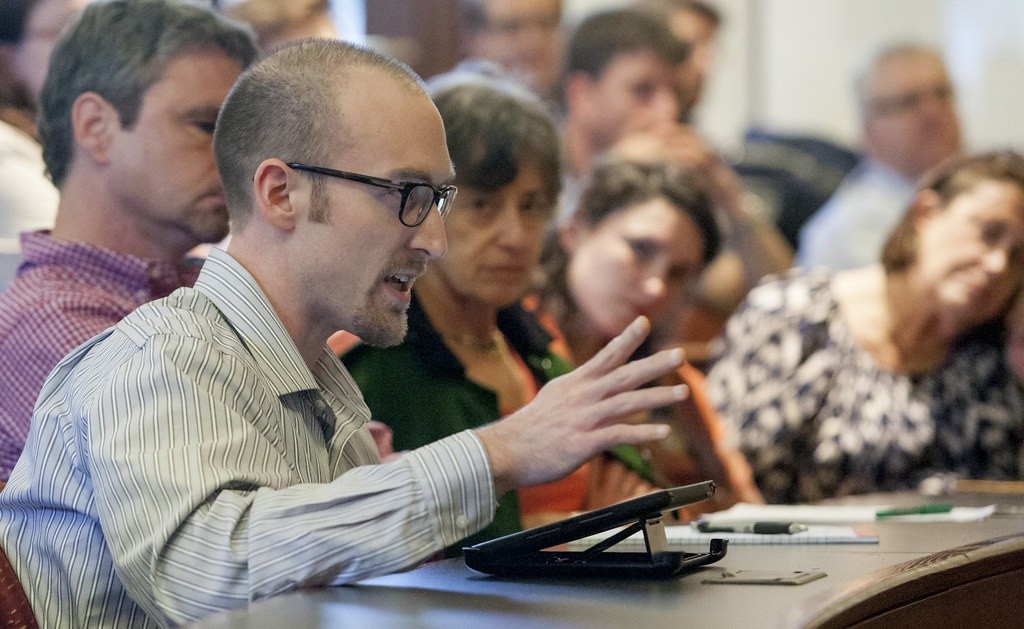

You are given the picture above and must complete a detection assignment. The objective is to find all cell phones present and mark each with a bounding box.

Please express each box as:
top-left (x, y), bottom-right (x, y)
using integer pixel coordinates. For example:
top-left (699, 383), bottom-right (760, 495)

top-left (470, 479), bottom-right (716, 555)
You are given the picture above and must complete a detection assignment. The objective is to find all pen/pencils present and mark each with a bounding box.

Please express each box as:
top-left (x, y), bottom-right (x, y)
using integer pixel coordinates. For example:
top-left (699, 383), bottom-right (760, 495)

top-left (690, 519), bottom-right (809, 535)
top-left (875, 503), bottom-right (954, 516)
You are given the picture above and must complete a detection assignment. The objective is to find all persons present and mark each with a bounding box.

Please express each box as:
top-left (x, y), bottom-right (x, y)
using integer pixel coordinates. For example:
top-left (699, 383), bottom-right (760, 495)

top-left (1, 0), bottom-right (1024, 629)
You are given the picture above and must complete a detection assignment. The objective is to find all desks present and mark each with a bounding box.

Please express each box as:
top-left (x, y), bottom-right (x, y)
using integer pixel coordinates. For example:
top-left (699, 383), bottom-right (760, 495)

top-left (182, 480), bottom-right (1023, 628)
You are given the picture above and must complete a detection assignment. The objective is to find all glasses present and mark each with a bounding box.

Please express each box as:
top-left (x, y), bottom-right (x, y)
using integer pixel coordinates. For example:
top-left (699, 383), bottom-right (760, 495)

top-left (252, 161), bottom-right (457, 228)
